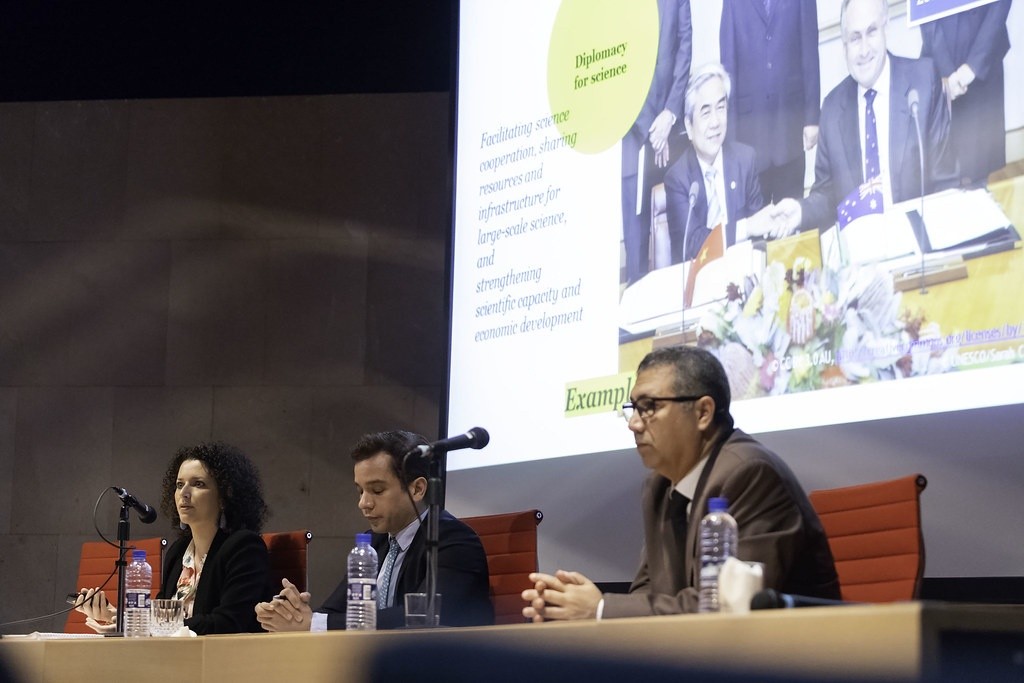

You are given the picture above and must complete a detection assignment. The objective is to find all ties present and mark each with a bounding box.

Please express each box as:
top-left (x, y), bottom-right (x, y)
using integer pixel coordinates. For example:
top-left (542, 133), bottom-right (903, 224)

top-left (863, 87), bottom-right (882, 213)
top-left (376, 537), bottom-right (400, 609)
top-left (671, 489), bottom-right (691, 593)
top-left (706, 168), bottom-right (723, 231)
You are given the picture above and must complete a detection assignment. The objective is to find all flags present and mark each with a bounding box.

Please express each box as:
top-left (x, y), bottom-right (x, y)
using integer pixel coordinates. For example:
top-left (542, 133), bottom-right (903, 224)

top-left (836, 174), bottom-right (884, 229)
top-left (685, 224), bottom-right (726, 305)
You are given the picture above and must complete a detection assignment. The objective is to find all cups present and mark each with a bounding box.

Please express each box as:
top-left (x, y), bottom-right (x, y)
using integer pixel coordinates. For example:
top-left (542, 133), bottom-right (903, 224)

top-left (404, 593), bottom-right (440, 629)
top-left (150, 599), bottom-right (184, 637)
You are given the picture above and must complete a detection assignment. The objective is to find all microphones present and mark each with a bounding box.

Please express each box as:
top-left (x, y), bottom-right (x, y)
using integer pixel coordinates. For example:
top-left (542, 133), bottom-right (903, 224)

top-left (652, 180), bottom-right (707, 351)
top-left (890, 90), bottom-right (971, 293)
top-left (112, 486), bottom-right (158, 524)
top-left (405, 428), bottom-right (490, 457)
top-left (748, 588), bottom-right (855, 609)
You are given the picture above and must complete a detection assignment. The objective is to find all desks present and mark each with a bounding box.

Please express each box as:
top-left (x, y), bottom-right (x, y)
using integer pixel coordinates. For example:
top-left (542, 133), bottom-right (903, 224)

top-left (0, 603), bottom-right (1024, 683)
top-left (614, 173), bottom-right (1024, 407)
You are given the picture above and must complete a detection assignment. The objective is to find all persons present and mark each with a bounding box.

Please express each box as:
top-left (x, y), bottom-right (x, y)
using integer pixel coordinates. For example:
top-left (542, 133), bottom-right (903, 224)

top-left (254, 432), bottom-right (494, 633)
top-left (75, 442), bottom-right (275, 633)
top-left (521, 346), bottom-right (843, 622)
top-left (623, 0), bottom-right (1013, 278)
top-left (664, 63), bottom-right (781, 260)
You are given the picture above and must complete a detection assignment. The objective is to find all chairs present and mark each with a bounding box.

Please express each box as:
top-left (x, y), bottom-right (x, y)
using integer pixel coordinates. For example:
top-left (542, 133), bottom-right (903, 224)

top-left (62, 538), bottom-right (167, 634)
top-left (455, 508), bottom-right (544, 625)
top-left (258, 531), bottom-right (313, 596)
top-left (806, 472), bottom-right (928, 606)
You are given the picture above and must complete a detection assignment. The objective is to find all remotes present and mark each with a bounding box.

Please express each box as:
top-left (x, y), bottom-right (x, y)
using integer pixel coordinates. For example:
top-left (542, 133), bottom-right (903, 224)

top-left (66, 592), bottom-right (109, 608)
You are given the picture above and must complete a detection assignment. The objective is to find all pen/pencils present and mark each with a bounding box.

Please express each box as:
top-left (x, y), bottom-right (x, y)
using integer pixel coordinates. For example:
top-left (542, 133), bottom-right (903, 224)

top-left (274, 595), bottom-right (288, 600)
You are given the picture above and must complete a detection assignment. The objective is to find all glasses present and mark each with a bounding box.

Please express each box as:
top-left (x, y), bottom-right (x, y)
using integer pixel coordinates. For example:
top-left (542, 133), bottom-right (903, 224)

top-left (622, 395), bottom-right (725, 422)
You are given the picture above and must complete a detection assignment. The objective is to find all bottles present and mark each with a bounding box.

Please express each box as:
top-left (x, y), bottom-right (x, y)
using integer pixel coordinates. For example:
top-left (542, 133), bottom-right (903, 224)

top-left (346, 535), bottom-right (376, 631)
top-left (124, 551), bottom-right (152, 638)
top-left (697, 495), bottom-right (738, 612)
